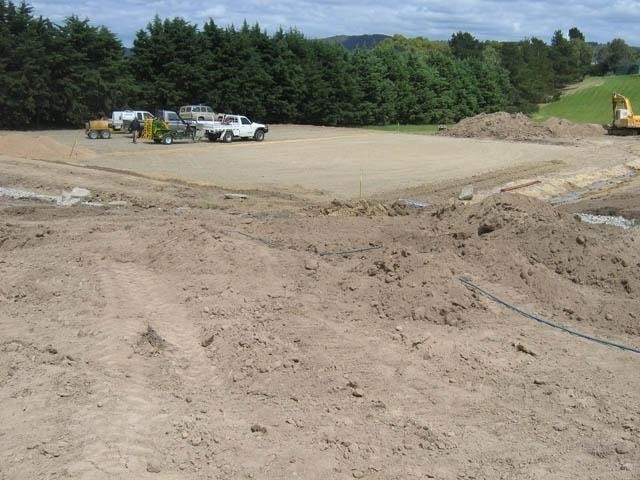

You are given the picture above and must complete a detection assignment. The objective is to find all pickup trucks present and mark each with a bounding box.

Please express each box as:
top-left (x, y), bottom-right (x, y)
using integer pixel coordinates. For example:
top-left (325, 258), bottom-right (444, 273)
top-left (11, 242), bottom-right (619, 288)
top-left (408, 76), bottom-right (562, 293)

top-left (85, 110), bottom-right (268, 144)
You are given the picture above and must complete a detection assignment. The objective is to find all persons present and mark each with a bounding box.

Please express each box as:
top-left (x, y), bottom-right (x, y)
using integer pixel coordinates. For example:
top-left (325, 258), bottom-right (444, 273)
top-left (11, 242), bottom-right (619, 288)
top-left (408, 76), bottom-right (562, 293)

top-left (129, 116), bottom-right (141, 144)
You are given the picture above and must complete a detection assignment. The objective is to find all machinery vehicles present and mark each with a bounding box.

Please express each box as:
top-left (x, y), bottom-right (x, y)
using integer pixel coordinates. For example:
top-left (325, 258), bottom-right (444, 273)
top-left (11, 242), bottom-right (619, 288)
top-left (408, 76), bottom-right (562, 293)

top-left (603, 92), bottom-right (640, 136)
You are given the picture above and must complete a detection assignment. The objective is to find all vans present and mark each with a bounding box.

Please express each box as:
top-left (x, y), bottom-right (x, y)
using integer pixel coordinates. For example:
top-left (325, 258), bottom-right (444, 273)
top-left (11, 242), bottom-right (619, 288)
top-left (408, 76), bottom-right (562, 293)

top-left (179, 106), bottom-right (216, 121)
